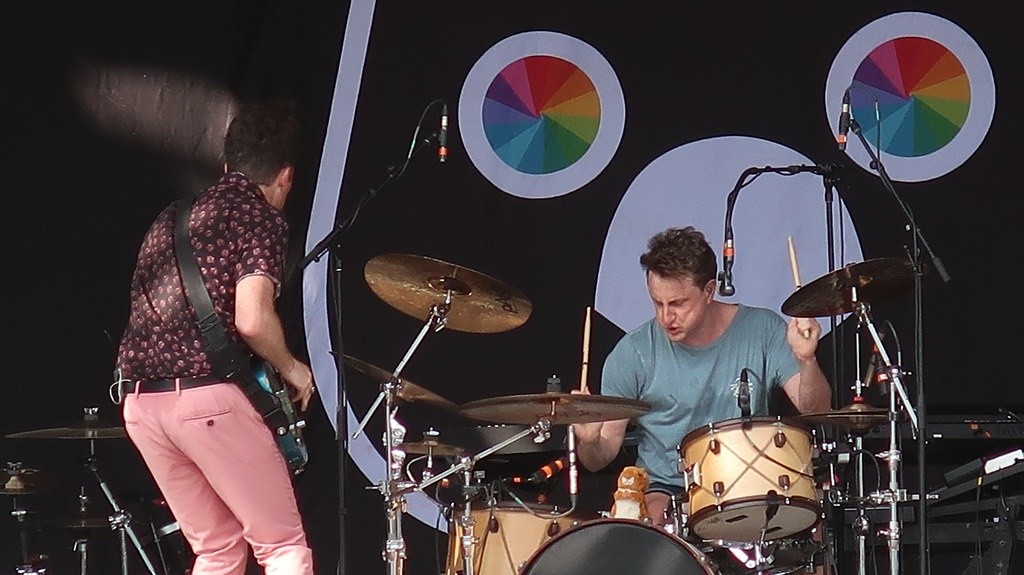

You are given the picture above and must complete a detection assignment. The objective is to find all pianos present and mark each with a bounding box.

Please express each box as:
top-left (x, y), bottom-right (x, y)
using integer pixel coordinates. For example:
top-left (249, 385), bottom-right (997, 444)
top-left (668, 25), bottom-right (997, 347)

top-left (863, 405), bottom-right (1024, 575)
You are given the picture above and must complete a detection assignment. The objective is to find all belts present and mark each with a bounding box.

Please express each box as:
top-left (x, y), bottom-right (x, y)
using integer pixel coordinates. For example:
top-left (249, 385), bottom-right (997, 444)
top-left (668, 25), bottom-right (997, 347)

top-left (121, 375), bottom-right (223, 393)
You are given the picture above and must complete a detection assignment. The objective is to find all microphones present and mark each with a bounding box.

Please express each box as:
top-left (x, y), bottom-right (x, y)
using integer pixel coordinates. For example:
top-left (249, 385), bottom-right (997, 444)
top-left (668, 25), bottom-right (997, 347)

top-left (438, 106), bottom-right (448, 163)
top-left (566, 425), bottom-right (578, 506)
top-left (737, 369), bottom-right (751, 409)
top-left (718, 228), bottom-right (735, 296)
top-left (839, 91), bottom-right (851, 151)
top-left (864, 322), bottom-right (889, 387)
top-left (533, 457), bottom-right (569, 483)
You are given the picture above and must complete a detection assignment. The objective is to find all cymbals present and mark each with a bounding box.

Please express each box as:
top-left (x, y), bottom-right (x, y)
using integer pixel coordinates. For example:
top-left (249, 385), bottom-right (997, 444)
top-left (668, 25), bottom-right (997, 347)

top-left (3, 419), bottom-right (129, 440)
top-left (447, 392), bottom-right (655, 427)
top-left (362, 251), bottom-right (534, 335)
top-left (790, 403), bottom-right (891, 436)
top-left (0, 466), bottom-right (40, 498)
top-left (327, 350), bottom-right (459, 415)
top-left (383, 441), bottom-right (464, 457)
top-left (779, 254), bottom-right (932, 319)
top-left (8, 513), bottom-right (115, 529)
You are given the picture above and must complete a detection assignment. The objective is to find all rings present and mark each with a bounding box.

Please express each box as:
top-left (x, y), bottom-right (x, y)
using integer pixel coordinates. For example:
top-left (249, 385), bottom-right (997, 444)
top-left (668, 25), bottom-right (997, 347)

top-left (312, 386), bottom-right (315, 391)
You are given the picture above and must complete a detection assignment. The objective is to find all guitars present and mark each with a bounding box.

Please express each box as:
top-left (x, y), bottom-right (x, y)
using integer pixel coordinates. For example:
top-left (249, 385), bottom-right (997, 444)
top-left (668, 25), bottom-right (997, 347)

top-left (241, 347), bottom-right (310, 477)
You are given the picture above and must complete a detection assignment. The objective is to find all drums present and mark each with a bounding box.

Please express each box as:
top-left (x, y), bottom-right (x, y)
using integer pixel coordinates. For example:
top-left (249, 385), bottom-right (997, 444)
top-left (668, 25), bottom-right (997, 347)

top-left (518, 518), bottom-right (722, 575)
top-left (678, 414), bottom-right (825, 543)
top-left (138, 517), bottom-right (197, 575)
top-left (444, 500), bottom-right (583, 575)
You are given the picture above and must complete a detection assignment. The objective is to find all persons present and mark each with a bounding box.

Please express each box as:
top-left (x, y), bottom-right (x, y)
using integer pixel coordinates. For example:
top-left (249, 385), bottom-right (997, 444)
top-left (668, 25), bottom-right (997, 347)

top-left (569, 226), bottom-right (831, 575)
top-left (114, 103), bottom-right (316, 575)
